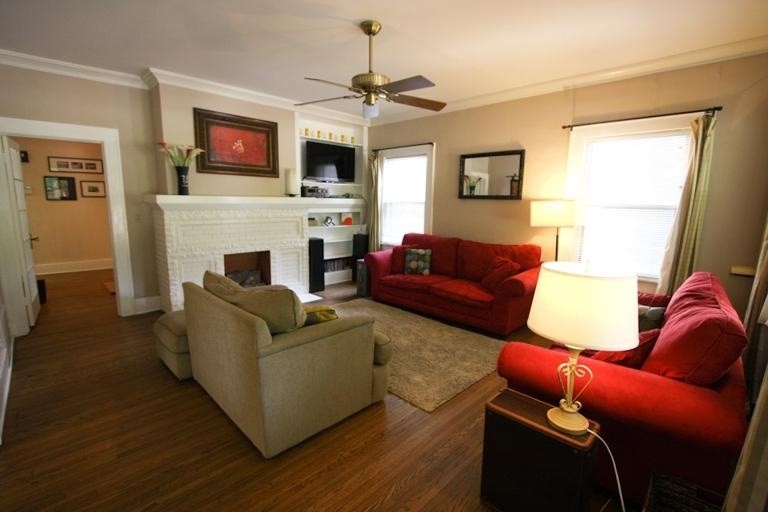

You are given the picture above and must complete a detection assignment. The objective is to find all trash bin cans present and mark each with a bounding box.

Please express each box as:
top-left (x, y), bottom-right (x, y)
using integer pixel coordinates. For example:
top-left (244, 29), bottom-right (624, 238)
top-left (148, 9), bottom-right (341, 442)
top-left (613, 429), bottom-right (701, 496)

top-left (356, 259), bottom-right (370, 298)
top-left (37, 279), bottom-right (47, 305)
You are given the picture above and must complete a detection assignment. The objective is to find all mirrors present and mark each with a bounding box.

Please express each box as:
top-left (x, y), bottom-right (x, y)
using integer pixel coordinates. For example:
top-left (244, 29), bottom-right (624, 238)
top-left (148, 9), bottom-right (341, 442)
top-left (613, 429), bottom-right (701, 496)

top-left (458, 150), bottom-right (524, 200)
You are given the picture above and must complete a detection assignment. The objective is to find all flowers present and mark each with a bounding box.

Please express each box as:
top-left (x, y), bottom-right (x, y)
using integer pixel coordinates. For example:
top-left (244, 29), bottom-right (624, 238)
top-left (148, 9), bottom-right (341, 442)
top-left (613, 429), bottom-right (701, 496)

top-left (156, 141), bottom-right (206, 166)
top-left (463, 174), bottom-right (484, 186)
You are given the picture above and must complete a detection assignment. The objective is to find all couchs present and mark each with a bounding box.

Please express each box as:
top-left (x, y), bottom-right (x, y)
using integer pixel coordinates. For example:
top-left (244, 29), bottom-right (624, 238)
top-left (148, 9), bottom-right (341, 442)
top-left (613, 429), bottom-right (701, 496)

top-left (363, 232), bottom-right (543, 337)
top-left (182, 282), bottom-right (393, 458)
top-left (496, 291), bottom-right (748, 511)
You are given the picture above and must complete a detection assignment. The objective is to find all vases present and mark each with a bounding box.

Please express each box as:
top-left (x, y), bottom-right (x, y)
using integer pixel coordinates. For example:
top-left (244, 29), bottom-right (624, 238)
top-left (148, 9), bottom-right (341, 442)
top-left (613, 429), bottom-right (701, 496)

top-left (174, 166), bottom-right (189, 194)
top-left (469, 185), bottom-right (476, 196)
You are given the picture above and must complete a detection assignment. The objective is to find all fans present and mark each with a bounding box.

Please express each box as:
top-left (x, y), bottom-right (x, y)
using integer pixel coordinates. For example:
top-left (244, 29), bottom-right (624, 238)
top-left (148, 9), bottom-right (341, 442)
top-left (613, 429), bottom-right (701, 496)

top-left (295, 20), bottom-right (447, 113)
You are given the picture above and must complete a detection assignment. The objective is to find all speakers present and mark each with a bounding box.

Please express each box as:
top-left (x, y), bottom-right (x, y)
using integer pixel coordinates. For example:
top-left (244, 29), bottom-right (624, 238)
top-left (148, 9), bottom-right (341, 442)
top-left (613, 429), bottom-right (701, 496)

top-left (352, 233), bottom-right (369, 282)
top-left (309, 237), bottom-right (324, 293)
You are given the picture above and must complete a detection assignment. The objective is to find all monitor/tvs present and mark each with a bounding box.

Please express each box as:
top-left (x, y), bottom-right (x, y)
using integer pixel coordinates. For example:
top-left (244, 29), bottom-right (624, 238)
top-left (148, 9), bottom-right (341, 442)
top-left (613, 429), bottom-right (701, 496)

top-left (305, 140), bottom-right (356, 183)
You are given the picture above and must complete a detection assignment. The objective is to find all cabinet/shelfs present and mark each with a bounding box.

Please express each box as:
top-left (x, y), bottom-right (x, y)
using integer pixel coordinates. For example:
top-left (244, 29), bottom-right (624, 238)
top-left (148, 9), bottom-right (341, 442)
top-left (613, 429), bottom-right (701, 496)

top-left (480, 388), bottom-right (600, 512)
top-left (308, 194), bottom-right (367, 285)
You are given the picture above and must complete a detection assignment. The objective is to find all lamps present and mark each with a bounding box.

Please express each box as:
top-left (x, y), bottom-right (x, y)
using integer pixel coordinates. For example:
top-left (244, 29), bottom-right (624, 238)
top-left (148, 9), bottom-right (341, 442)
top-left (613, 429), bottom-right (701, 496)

top-left (529, 200), bottom-right (577, 261)
top-left (361, 93), bottom-right (381, 117)
top-left (527, 261), bottom-right (640, 437)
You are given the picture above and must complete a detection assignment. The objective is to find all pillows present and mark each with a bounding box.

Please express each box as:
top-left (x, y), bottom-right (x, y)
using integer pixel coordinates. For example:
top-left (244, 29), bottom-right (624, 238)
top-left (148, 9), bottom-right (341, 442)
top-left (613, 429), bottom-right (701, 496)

top-left (478, 256), bottom-right (522, 294)
top-left (638, 305), bottom-right (665, 332)
top-left (392, 247), bottom-right (433, 273)
top-left (302, 306), bottom-right (338, 323)
top-left (588, 329), bottom-right (659, 370)
top-left (643, 271), bottom-right (750, 386)
top-left (202, 270), bottom-right (307, 334)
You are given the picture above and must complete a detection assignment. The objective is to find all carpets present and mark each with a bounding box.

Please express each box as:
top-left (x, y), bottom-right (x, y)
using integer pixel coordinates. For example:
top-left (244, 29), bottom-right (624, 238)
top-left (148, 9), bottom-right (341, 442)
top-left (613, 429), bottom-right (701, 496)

top-left (334, 298), bottom-right (510, 413)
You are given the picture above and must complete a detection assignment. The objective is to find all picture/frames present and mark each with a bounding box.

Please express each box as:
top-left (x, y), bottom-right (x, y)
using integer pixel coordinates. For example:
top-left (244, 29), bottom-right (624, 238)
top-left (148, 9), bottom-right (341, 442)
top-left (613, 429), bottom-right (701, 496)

top-left (192, 107), bottom-right (279, 178)
top-left (44, 156), bottom-right (105, 201)
